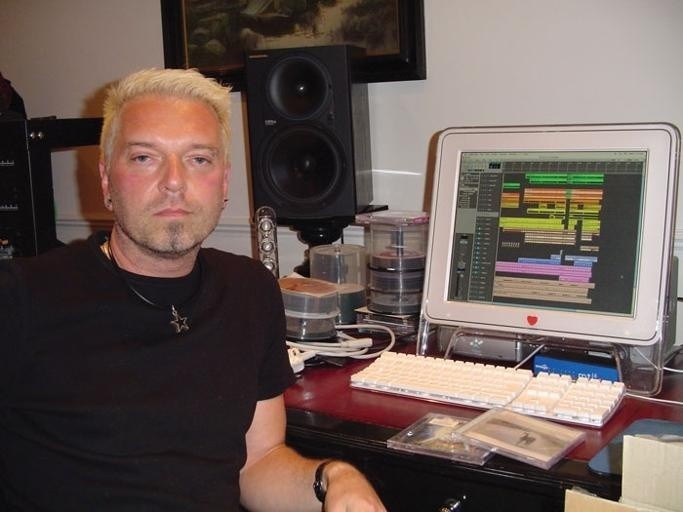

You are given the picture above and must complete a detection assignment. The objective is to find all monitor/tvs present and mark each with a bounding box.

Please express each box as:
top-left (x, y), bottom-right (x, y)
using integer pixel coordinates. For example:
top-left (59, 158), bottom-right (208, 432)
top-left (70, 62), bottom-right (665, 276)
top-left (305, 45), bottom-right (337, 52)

top-left (416, 123), bottom-right (681, 396)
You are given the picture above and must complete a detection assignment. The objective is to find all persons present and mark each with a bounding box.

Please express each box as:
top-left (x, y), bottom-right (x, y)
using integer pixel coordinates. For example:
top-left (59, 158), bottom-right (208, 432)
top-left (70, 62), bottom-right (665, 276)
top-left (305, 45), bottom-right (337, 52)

top-left (0, 64), bottom-right (386, 512)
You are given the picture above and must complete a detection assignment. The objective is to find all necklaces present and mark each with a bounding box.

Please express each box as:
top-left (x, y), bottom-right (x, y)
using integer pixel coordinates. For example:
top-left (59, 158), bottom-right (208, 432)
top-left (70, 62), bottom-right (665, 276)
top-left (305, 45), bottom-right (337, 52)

top-left (105, 232), bottom-right (207, 335)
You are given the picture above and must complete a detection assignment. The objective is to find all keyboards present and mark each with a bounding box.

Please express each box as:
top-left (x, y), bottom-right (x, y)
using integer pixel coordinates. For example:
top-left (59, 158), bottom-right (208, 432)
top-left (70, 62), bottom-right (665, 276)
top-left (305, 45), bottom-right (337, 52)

top-left (350, 350), bottom-right (625, 426)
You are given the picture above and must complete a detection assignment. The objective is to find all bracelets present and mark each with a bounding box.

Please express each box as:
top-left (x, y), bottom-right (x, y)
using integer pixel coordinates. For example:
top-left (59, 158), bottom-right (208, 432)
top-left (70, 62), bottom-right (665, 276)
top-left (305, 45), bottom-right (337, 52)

top-left (314, 459), bottom-right (337, 504)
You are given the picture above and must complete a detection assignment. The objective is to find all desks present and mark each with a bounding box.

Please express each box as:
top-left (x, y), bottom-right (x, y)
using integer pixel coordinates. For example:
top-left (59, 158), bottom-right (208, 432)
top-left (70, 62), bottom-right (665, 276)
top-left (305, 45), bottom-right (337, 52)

top-left (284, 333), bottom-right (683, 512)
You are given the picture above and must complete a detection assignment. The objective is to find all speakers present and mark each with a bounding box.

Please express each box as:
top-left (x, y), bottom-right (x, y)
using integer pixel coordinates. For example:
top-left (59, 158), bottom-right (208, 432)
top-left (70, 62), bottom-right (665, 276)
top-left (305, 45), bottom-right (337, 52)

top-left (244, 45), bottom-right (373, 226)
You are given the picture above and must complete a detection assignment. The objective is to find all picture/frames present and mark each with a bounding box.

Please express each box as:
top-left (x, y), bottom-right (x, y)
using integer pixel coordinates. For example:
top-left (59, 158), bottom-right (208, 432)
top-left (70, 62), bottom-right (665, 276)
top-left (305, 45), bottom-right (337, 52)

top-left (159, 0), bottom-right (428, 94)
